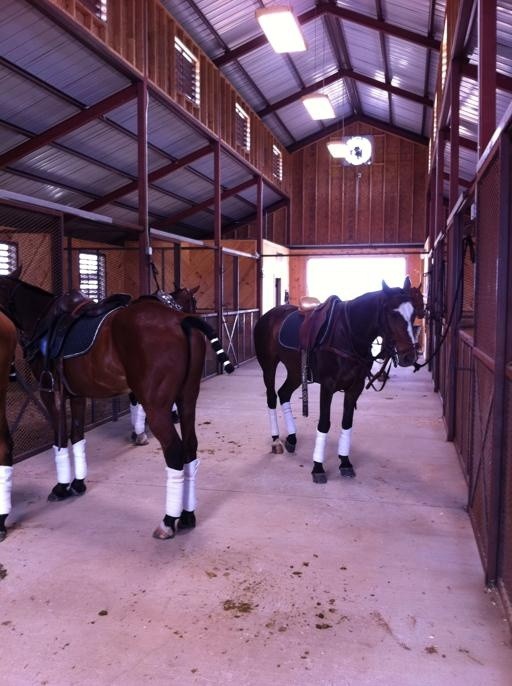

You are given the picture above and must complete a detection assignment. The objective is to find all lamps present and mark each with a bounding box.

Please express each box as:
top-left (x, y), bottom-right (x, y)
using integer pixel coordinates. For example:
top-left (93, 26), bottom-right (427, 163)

top-left (326, 80), bottom-right (351, 158)
top-left (299, 14), bottom-right (336, 121)
top-left (255, 0), bottom-right (306, 55)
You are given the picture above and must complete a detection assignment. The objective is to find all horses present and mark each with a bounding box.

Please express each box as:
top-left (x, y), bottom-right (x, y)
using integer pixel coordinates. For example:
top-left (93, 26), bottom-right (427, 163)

top-left (0, 263), bottom-right (234, 542)
top-left (128, 282), bottom-right (200, 445)
top-left (411, 282), bottom-right (425, 319)
top-left (254, 276), bottom-right (418, 483)
